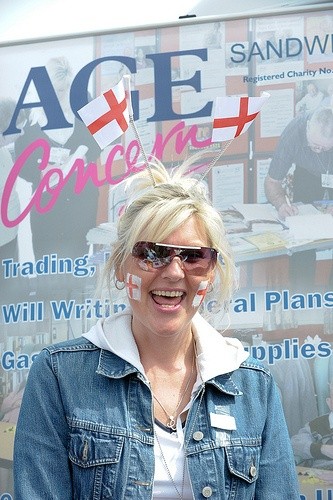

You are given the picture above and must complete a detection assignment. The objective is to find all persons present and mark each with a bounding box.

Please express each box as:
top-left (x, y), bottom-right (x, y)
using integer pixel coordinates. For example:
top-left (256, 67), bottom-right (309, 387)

top-left (289, 378), bottom-right (332, 470)
top-left (264, 79), bottom-right (333, 216)
top-left (10, 64), bottom-right (109, 276)
top-left (13, 182), bottom-right (300, 500)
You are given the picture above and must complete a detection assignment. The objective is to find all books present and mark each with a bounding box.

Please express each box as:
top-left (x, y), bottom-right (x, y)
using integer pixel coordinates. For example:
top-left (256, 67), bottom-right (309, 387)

top-left (217, 201), bottom-right (332, 259)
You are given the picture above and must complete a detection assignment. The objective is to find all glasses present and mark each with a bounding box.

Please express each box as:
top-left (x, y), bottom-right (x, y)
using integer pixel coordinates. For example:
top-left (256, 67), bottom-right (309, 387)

top-left (129, 241), bottom-right (219, 276)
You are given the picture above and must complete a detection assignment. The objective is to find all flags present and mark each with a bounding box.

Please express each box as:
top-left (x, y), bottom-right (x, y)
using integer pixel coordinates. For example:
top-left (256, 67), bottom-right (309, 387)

top-left (76, 74), bottom-right (133, 150)
top-left (210, 90), bottom-right (271, 143)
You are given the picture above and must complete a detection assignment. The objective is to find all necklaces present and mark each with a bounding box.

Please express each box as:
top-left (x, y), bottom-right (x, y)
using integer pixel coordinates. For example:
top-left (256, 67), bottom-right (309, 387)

top-left (149, 345), bottom-right (205, 499)
